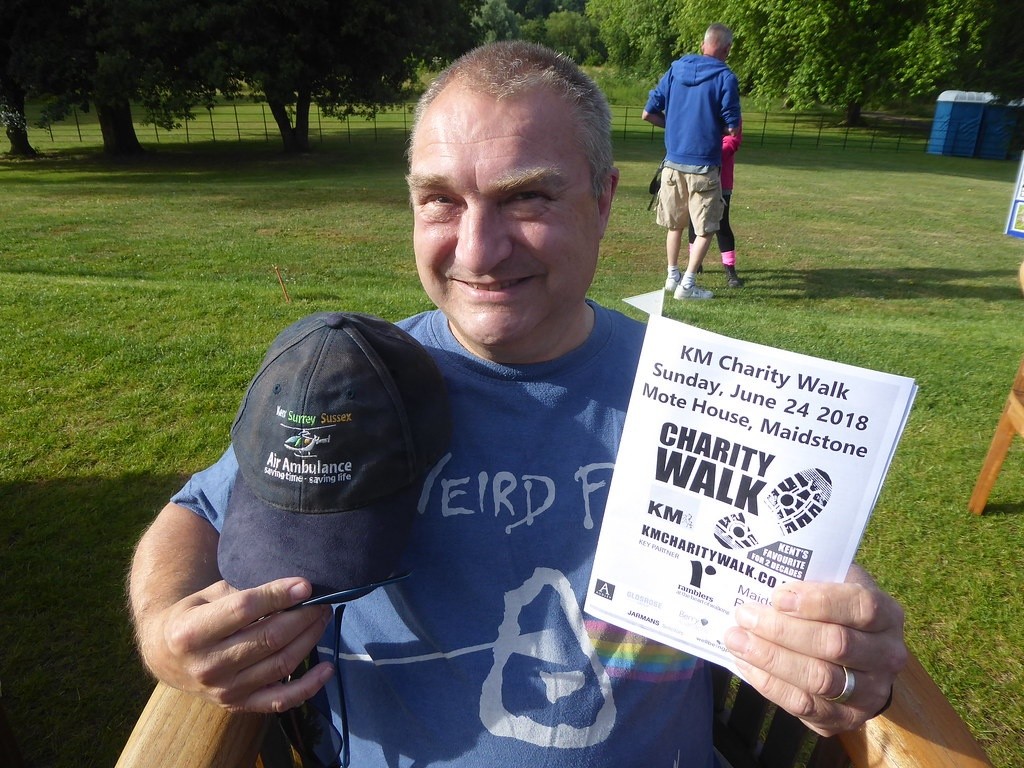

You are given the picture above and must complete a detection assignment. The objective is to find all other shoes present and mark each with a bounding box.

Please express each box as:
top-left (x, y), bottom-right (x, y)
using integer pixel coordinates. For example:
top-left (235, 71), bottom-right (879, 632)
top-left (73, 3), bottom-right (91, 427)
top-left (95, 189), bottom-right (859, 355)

top-left (725, 272), bottom-right (741, 288)
top-left (697, 264), bottom-right (703, 275)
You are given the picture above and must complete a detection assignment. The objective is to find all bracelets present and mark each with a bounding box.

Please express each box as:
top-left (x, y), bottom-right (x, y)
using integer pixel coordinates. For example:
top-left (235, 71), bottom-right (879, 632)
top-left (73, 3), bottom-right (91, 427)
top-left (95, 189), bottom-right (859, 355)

top-left (874, 685), bottom-right (895, 716)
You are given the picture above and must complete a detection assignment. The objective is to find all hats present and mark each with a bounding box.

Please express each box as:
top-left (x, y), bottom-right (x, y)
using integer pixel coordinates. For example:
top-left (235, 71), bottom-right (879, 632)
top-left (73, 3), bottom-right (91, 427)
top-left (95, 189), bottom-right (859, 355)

top-left (216, 309), bottom-right (455, 610)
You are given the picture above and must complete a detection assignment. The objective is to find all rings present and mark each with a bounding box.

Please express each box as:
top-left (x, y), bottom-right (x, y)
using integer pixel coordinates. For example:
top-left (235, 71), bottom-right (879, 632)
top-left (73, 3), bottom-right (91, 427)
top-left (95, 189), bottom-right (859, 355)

top-left (823, 666), bottom-right (855, 704)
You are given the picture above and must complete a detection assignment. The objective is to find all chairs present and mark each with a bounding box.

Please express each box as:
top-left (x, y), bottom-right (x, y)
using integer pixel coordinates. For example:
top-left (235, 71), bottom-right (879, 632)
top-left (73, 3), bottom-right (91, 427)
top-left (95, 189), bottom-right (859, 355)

top-left (114, 645), bottom-right (993, 768)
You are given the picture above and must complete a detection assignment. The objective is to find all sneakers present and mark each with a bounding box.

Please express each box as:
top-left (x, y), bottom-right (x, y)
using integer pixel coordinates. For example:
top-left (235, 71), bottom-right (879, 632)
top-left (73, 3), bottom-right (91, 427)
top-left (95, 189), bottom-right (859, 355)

top-left (664, 273), bottom-right (680, 291)
top-left (672, 283), bottom-right (715, 301)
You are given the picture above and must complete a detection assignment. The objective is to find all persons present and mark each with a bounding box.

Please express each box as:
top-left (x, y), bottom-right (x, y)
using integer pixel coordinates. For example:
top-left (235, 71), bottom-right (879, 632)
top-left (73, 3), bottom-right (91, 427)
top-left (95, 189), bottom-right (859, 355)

top-left (642, 24), bottom-right (743, 301)
top-left (127, 40), bottom-right (910, 768)
